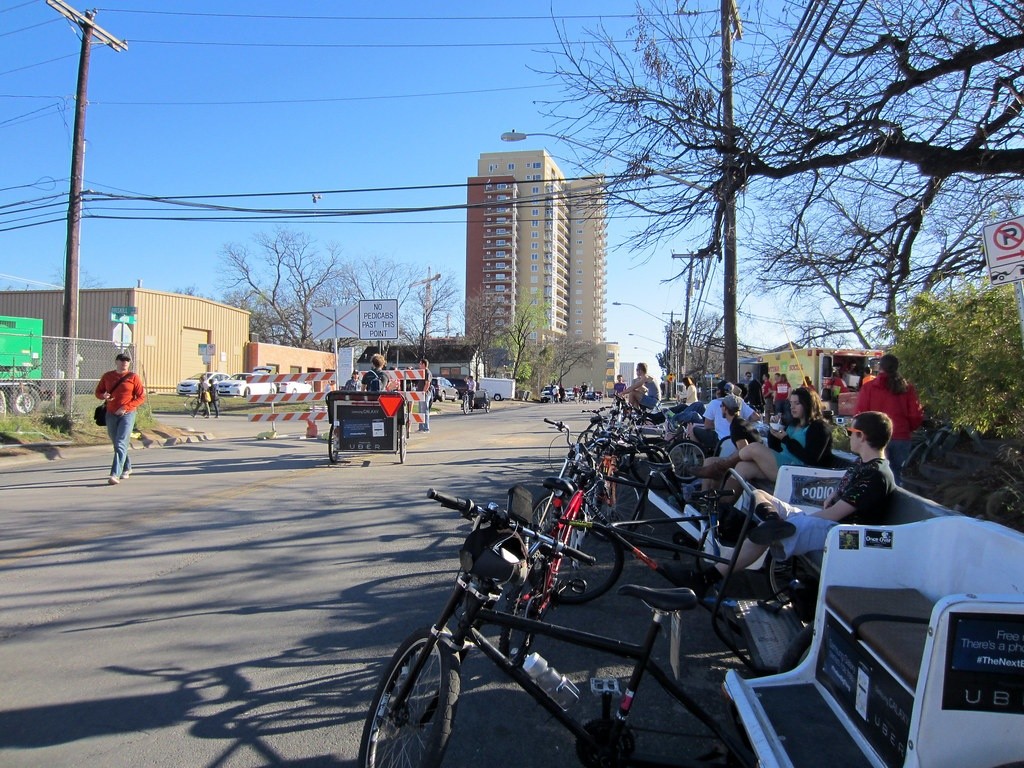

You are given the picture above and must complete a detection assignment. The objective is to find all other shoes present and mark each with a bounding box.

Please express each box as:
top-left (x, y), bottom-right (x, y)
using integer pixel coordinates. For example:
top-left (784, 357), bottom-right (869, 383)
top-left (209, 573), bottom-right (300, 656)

top-left (109, 476), bottom-right (120, 485)
top-left (120, 469), bottom-right (132, 478)
top-left (416, 429), bottom-right (430, 433)
top-left (190, 413), bottom-right (196, 418)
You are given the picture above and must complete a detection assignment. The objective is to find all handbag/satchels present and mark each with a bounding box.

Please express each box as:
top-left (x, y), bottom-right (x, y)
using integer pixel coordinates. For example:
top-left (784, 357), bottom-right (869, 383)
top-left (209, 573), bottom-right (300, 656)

top-left (94, 404), bottom-right (106, 426)
top-left (203, 391), bottom-right (211, 402)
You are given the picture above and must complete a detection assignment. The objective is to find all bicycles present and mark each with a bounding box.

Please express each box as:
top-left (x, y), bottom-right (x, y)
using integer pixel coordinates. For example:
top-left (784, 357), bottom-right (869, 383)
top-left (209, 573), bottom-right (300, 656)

top-left (185, 395), bottom-right (226, 413)
top-left (461, 390), bottom-right (490, 414)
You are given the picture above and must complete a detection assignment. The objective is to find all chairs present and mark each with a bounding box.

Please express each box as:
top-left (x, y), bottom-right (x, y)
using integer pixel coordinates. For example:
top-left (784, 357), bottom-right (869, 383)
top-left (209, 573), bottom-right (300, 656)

top-left (236, 375), bottom-right (246, 380)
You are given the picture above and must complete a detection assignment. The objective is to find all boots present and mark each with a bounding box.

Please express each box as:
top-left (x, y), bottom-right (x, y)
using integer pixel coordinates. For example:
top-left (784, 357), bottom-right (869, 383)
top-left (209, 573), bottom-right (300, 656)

top-left (663, 561), bottom-right (723, 598)
top-left (688, 451), bottom-right (741, 480)
top-left (749, 502), bottom-right (796, 545)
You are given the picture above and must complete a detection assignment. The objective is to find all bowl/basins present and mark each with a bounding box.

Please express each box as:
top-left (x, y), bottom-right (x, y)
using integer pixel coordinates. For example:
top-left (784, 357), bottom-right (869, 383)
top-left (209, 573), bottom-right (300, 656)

top-left (769, 423), bottom-right (785, 432)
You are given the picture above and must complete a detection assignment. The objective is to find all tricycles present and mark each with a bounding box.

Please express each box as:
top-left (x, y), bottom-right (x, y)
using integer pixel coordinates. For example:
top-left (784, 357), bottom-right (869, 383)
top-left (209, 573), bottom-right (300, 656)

top-left (500, 404), bottom-right (956, 666)
top-left (357, 488), bottom-right (1024, 768)
top-left (326, 391), bottom-right (409, 465)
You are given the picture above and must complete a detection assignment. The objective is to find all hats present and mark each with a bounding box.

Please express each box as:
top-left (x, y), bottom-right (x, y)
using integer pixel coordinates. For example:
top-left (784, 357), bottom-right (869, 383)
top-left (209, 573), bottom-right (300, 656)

top-left (717, 380), bottom-right (734, 396)
top-left (722, 395), bottom-right (742, 411)
top-left (116, 354), bottom-right (131, 360)
top-left (353, 370), bottom-right (359, 374)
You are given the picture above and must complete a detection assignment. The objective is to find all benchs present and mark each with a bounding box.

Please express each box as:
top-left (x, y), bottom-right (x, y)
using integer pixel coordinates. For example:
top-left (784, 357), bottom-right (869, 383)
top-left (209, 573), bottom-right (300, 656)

top-left (809, 511), bottom-right (1024, 768)
top-left (729, 434), bottom-right (850, 518)
top-left (326, 390), bottom-right (408, 426)
top-left (786, 472), bottom-right (946, 629)
top-left (474, 388), bottom-right (488, 399)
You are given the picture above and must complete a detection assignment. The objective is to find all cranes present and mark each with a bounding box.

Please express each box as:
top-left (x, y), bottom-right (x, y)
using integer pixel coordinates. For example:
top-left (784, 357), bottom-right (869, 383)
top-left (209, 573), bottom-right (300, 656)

top-left (409, 266), bottom-right (441, 338)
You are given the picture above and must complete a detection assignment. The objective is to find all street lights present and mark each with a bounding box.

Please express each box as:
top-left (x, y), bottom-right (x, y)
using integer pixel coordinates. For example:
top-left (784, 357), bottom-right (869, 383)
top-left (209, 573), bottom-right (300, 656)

top-left (502, 131), bottom-right (737, 386)
top-left (612, 302), bottom-right (673, 399)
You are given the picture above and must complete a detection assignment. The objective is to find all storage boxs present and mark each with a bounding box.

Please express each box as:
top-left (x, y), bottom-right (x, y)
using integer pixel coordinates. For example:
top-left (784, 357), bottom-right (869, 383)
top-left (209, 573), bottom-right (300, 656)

top-left (844, 373), bottom-right (861, 386)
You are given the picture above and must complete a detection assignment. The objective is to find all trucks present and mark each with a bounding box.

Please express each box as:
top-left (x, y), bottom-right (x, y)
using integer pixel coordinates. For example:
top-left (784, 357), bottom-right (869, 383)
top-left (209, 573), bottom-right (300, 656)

top-left (476, 378), bottom-right (516, 401)
top-left (758, 348), bottom-right (883, 400)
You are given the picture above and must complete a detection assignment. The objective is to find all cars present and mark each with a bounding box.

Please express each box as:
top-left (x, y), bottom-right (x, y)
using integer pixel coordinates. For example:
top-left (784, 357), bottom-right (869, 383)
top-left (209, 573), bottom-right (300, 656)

top-left (706, 389), bottom-right (717, 400)
top-left (563, 388), bottom-right (603, 401)
top-left (216, 373), bottom-right (277, 398)
top-left (176, 372), bottom-right (231, 397)
top-left (275, 380), bottom-right (313, 394)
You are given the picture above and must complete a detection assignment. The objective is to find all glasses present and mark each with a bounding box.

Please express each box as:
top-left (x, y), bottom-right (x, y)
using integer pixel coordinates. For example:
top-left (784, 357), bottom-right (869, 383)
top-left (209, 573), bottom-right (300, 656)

top-left (845, 427), bottom-right (864, 437)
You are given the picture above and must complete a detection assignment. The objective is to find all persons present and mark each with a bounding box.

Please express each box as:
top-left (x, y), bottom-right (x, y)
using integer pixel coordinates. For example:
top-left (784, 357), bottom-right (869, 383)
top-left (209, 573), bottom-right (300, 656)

top-left (389, 366), bottom-right (419, 413)
top-left (415, 359), bottom-right (432, 433)
top-left (645, 381), bottom-right (895, 590)
top-left (675, 376), bottom-right (699, 406)
top-left (324, 380), bottom-right (335, 401)
top-left (614, 363), bottom-right (659, 410)
top-left (461, 376), bottom-right (476, 410)
top-left (361, 354), bottom-right (390, 391)
top-left (572, 382), bottom-right (588, 404)
top-left (95, 353), bottom-right (146, 484)
top-left (201, 379), bottom-right (219, 418)
top-left (738, 354), bottom-right (925, 487)
top-left (550, 384), bottom-right (565, 404)
top-left (344, 369), bottom-right (361, 390)
top-left (190, 375), bottom-right (211, 418)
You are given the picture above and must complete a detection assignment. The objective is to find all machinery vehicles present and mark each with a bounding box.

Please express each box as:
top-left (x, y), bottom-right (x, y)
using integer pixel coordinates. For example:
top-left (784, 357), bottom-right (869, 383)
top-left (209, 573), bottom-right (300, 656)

top-left (0, 316), bottom-right (43, 415)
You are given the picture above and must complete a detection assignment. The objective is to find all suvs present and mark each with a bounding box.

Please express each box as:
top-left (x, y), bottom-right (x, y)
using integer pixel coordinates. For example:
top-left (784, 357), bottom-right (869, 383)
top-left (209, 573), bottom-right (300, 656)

top-left (415, 377), bottom-right (459, 403)
top-left (446, 377), bottom-right (470, 398)
top-left (541, 385), bottom-right (560, 403)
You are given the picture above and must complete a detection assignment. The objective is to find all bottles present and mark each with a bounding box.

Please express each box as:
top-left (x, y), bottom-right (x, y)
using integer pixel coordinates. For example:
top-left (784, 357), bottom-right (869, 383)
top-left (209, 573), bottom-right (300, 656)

top-left (523, 652), bottom-right (580, 712)
